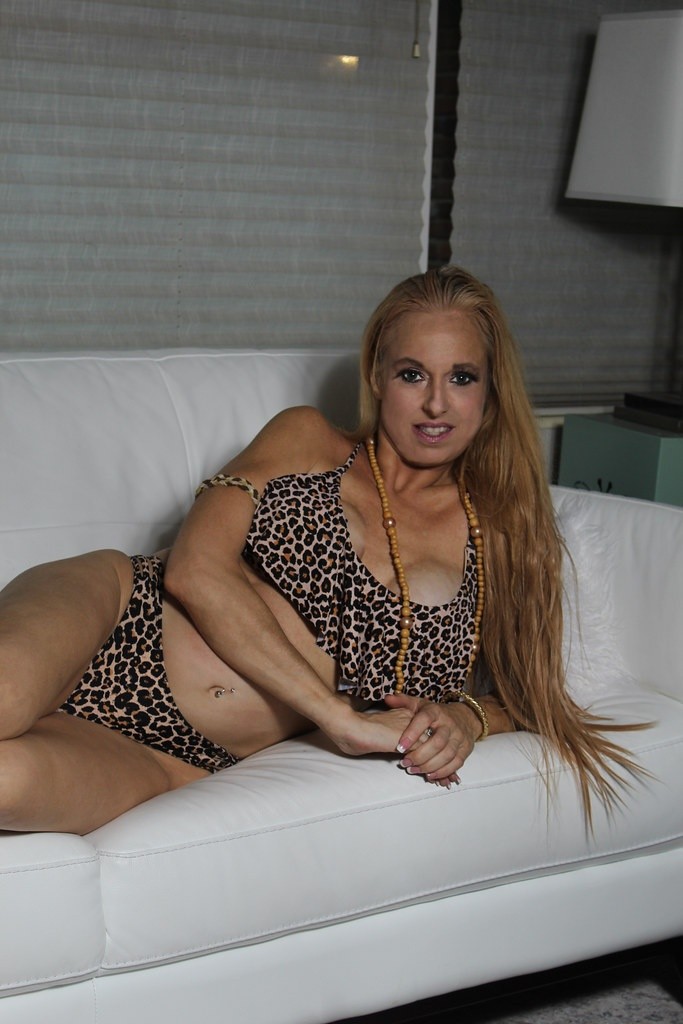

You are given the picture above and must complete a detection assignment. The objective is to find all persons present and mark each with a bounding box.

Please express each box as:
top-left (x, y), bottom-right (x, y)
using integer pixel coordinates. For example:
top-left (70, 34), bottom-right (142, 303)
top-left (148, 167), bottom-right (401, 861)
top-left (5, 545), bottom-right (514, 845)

top-left (0, 267), bottom-right (661, 842)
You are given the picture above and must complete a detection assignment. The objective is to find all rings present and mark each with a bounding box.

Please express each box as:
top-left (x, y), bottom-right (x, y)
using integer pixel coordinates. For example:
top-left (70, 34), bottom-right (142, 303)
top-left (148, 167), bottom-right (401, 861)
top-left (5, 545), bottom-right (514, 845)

top-left (427, 727), bottom-right (433, 736)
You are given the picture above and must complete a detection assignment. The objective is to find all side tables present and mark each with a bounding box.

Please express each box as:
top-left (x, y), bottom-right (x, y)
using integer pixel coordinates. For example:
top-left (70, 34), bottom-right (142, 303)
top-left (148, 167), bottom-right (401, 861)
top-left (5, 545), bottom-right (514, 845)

top-left (557, 413), bottom-right (683, 508)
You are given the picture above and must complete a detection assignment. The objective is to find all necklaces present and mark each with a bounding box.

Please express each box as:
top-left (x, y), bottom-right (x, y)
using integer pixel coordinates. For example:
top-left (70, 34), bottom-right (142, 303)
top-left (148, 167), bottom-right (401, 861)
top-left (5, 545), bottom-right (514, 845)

top-left (364, 432), bottom-right (486, 699)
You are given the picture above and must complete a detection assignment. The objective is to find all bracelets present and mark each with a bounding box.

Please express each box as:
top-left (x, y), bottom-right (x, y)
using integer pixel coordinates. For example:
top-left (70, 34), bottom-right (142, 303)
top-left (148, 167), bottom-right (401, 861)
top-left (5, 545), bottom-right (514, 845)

top-left (454, 688), bottom-right (489, 742)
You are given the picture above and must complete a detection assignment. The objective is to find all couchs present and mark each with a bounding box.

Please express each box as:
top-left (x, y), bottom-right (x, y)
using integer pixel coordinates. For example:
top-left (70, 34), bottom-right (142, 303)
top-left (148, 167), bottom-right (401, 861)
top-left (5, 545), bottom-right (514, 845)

top-left (0, 348), bottom-right (683, 1024)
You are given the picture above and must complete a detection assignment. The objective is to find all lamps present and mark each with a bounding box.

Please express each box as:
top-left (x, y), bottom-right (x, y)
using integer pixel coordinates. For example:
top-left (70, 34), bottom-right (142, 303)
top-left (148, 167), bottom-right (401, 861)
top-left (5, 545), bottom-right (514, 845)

top-left (564, 11), bottom-right (683, 434)
